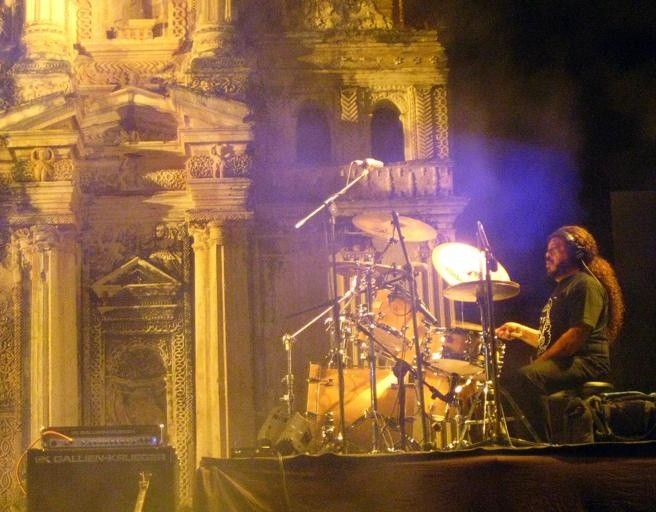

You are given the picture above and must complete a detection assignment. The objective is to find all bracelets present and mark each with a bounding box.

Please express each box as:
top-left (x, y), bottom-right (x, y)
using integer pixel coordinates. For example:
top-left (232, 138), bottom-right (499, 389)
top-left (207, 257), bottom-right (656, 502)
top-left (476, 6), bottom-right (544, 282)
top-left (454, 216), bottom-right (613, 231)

top-left (540, 355), bottom-right (546, 362)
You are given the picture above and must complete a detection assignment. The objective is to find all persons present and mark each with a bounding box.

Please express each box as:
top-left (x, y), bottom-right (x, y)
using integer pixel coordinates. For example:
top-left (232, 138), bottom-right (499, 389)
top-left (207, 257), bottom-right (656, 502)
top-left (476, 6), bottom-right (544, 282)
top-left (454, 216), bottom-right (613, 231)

top-left (494, 226), bottom-right (626, 442)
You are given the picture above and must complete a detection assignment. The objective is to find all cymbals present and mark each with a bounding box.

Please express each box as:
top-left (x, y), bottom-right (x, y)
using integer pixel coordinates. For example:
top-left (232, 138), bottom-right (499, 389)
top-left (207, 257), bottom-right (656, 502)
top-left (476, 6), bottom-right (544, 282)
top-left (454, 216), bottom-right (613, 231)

top-left (431, 242), bottom-right (510, 287)
top-left (353, 212), bottom-right (437, 244)
top-left (328, 258), bottom-right (407, 279)
top-left (444, 279), bottom-right (519, 303)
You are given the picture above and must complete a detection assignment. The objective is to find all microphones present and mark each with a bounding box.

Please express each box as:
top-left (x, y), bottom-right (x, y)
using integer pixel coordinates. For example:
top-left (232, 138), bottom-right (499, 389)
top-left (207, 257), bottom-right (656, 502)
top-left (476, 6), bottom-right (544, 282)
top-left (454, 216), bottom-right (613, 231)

top-left (354, 157), bottom-right (385, 170)
top-left (477, 220), bottom-right (498, 272)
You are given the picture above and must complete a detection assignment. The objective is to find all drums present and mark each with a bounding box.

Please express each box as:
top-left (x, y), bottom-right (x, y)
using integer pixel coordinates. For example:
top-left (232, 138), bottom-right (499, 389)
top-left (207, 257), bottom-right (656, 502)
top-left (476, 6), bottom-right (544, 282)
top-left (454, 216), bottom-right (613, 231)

top-left (302, 362), bottom-right (416, 453)
top-left (424, 359), bottom-right (486, 421)
top-left (424, 328), bottom-right (498, 380)
top-left (363, 284), bottom-right (426, 356)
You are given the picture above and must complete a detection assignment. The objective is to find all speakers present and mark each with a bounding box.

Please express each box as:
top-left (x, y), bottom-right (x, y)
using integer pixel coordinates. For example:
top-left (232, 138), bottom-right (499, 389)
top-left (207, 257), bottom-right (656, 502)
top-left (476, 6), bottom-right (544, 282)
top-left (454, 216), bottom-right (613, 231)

top-left (27, 448), bottom-right (176, 512)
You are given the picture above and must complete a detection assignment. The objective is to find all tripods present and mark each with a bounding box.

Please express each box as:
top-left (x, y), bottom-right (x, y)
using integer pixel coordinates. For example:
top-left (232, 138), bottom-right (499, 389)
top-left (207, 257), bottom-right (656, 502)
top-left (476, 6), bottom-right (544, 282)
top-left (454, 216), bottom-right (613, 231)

top-left (354, 318), bottom-right (446, 452)
top-left (325, 203), bottom-right (362, 454)
top-left (339, 278), bottom-right (423, 451)
top-left (390, 210), bottom-right (441, 452)
top-left (454, 248), bottom-right (544, 447)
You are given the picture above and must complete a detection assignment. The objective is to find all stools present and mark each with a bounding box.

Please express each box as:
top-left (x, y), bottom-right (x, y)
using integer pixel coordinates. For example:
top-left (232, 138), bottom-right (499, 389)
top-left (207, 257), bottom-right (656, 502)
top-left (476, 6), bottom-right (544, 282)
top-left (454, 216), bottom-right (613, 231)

top-left (539, 380), bottom-right (615, 443)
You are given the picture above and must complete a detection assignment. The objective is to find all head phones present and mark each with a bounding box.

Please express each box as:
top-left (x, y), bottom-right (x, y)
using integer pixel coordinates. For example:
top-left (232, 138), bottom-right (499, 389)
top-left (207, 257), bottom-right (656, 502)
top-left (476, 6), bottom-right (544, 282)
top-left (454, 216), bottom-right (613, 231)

top-left (547, 231), bottom-right (589, 266)
